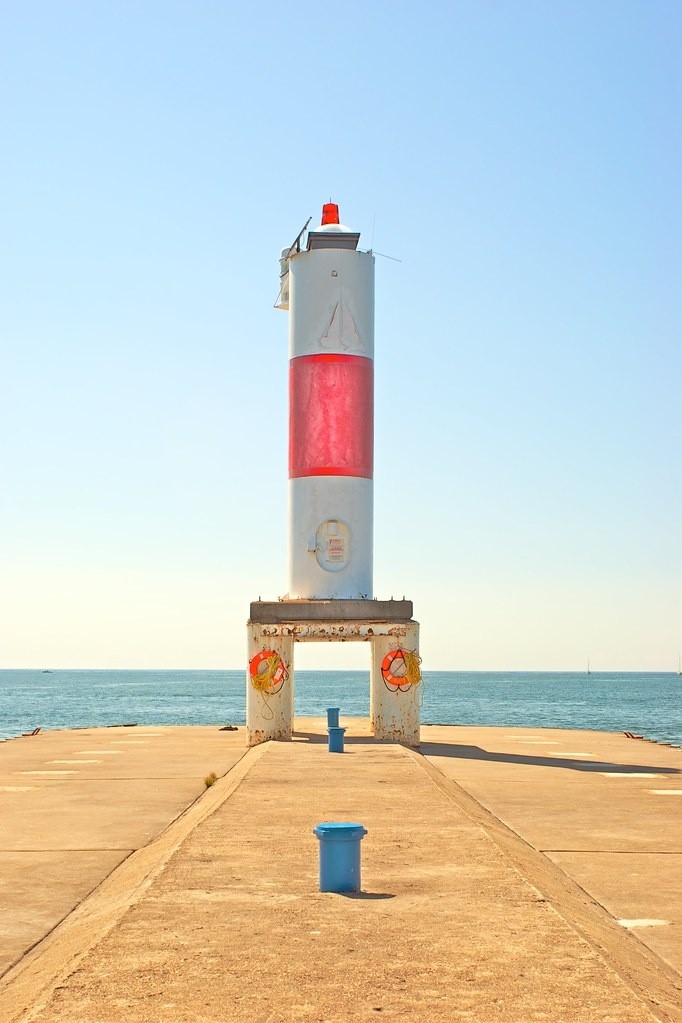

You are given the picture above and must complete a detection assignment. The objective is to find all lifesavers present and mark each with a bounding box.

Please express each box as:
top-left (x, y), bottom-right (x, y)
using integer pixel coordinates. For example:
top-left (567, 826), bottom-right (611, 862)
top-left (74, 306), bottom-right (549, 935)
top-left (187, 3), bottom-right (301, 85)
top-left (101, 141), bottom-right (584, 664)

top-left (249, 651), bottom-right (285, 687)
top-left (381, 650), bottom-right (413, 686)
